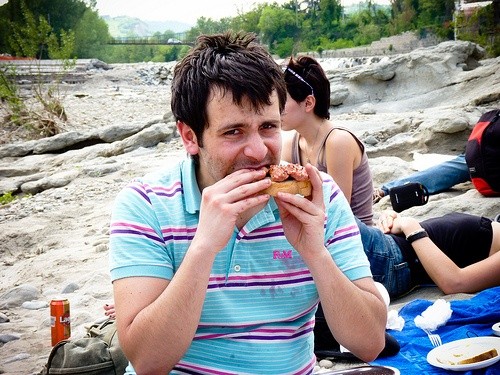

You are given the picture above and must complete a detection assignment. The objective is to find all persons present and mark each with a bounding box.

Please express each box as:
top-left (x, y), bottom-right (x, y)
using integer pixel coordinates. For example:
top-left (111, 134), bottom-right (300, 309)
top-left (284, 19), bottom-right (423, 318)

top-left (279, 53), bottom-right (373, 228)
top-left (373, 154), bottom-right (471, 203)
top-left (107, 33), bottom-right (389, 375)
top-left (354, 212), bottom-right (500, 301)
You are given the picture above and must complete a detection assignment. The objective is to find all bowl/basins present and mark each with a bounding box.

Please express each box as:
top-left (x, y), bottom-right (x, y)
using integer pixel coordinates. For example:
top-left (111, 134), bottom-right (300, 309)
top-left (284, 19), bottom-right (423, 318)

top-left (315, 364), bottom-right (401, 375)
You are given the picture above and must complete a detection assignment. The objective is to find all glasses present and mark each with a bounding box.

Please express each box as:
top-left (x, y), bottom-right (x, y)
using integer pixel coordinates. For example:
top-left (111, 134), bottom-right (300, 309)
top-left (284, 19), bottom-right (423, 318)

top-left (280, 62), bottom-right (314, 97)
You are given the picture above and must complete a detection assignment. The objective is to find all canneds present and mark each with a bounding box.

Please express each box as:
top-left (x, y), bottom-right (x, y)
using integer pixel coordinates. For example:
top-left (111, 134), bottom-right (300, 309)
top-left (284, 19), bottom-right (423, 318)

top-left (50, 298), bottom-right (71, 347)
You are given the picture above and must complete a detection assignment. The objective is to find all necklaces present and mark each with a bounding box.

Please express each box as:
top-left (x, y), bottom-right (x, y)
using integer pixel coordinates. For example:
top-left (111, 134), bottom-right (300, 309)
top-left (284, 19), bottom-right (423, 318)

top-left (301, 118), bottom-right (323, 164)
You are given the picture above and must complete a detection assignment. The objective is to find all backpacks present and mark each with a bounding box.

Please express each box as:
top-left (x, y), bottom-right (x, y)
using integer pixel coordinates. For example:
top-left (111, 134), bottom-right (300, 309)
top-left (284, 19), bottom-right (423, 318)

top-left (41, 318), bottom-right (129, 375)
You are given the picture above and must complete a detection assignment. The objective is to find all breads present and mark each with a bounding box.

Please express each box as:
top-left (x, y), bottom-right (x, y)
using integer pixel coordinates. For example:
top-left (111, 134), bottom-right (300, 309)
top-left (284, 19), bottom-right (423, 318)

top-left (437, 343), bottom-right (498, 365)
top-left (262, 178), bottom-right (312, 196)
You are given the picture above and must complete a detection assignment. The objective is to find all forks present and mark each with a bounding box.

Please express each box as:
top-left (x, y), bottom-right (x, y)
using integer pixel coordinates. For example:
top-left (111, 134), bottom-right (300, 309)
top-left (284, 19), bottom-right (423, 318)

top-left (421, 328), bottom-right (442, 347)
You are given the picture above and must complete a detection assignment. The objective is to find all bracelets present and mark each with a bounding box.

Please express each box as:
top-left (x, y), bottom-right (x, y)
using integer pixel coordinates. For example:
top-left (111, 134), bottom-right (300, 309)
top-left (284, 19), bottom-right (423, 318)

top-left (405, 229), bottom-right (428, 243)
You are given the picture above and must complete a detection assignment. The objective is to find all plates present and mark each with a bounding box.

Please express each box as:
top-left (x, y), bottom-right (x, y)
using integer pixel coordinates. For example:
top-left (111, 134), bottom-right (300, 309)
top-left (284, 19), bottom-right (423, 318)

top-left (426, 336), bottom-right (500, 371)
